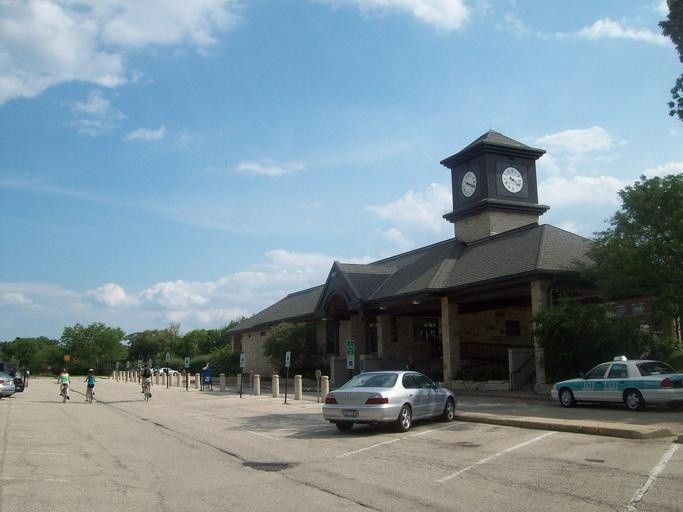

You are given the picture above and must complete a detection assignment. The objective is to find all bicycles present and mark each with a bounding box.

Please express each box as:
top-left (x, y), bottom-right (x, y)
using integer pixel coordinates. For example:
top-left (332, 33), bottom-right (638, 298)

top-left (59, 381), bottom-right (70, 402)
top-left (85, 380), bottom-right (96, 403)
top-left (140, 376), bottom-right (150, 402)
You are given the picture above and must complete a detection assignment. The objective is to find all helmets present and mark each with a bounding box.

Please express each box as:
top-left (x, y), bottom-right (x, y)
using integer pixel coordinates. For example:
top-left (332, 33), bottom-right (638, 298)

top-left (89, 369), bottom-right (93, 371)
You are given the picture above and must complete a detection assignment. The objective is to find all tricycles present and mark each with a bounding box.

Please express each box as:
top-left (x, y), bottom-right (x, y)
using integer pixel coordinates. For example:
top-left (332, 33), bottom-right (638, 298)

top-left (201, 371), bottom-right (212, 391)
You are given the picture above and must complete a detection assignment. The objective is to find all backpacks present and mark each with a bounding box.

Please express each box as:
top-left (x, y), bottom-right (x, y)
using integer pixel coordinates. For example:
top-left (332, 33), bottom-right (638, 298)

top-left (144, 369), bottom-right (151, 378)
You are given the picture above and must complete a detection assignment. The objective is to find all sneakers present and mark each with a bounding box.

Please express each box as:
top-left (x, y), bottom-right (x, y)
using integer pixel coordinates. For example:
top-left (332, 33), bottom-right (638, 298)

top-left (142, 391), bottom-right (144, 393)
top-left (67, 396), bottom-right (69, 399)
top-left (86, 400), bottom-right (88, 401)
top-left (149, 394), bottom-right (151, 398)
top-left (60, 394), bottom-right (62, 395)
top-left (93, 393), bottom-right (95, 395)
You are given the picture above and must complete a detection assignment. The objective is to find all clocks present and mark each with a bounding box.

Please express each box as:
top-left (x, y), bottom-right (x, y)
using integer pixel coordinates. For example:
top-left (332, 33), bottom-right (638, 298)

top-left (501, 166), bottom-right (525, 194)
top-left (461, 171), bottom-right (477, 198)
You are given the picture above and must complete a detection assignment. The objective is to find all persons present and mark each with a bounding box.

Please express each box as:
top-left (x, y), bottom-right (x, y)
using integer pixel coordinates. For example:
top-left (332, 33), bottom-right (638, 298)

top-left (137, 364), bottom-right (152, 398)
top-left (406, 357), bottom-right (415, 370)
top-left (57, 368), bottom-right (71, 399)
top-left (84, 368), bottom-right (96, 401)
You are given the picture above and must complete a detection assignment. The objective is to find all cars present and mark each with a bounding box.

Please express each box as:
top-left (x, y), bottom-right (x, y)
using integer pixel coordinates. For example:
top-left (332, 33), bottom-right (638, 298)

top-left (149, 366), bottom-right (178, 376)
top-left (551, 359), bottom-right (683, 410)
top-left (0, 372), bottom-right (25, 398)
top-left (322, 369), bottom-right (457, 432)
